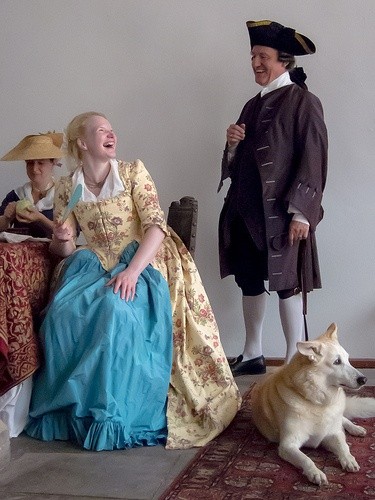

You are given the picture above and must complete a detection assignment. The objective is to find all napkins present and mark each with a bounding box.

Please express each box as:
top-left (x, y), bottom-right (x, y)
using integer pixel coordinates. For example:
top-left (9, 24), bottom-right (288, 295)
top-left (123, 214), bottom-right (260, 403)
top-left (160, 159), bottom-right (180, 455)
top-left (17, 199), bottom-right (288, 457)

top-left (0, 231), bottom-right (53, 244)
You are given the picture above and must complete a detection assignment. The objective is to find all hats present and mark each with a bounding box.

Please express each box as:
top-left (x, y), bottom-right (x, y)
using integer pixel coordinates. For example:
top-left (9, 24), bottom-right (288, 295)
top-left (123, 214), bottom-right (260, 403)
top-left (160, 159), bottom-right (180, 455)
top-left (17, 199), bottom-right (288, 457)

top-left (0, 130), bottom-right (67, 160)
top-left (245, 19), bottom-right (316, 56)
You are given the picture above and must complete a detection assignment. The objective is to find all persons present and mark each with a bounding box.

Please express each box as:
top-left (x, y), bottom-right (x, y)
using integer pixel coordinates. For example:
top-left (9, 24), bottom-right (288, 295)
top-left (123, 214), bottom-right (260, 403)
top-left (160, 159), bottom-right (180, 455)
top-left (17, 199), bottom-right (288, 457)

top-left (0, 130), bottom-right (82, 243)
top-left (21, 110), bottom-right (173, 450)
top-left (216, 17), bottom-right (329, 379)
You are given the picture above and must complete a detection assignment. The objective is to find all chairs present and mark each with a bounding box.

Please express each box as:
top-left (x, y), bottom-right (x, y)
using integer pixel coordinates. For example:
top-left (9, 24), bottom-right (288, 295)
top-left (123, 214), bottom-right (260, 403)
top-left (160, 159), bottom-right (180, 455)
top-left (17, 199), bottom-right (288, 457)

top-left (167, 196), bottom-right (198, 260)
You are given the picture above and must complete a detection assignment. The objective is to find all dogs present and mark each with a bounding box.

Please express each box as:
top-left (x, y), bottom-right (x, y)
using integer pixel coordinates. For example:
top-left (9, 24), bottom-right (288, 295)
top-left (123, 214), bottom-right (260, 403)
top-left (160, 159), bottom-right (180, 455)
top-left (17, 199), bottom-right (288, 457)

top-left (251, 322), bottom-right (375, 487)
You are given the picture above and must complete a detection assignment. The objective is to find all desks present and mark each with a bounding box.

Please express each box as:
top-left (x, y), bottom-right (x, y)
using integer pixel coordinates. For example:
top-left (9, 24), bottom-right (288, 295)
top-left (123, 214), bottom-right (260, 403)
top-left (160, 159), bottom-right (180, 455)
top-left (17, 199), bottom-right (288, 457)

top-left (0, 239), bottom-right (51, 438)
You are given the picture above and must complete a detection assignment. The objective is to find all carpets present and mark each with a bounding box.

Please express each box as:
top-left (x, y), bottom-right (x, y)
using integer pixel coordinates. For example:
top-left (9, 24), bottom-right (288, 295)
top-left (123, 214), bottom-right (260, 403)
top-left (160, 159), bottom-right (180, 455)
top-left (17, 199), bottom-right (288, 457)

top-left (157, 381), bottom-right (375, 500)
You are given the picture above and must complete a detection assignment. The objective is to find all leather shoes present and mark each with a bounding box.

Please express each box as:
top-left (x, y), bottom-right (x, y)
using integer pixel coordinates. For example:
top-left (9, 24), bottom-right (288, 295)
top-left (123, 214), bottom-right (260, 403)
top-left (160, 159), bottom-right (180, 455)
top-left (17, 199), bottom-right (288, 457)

top-left (228, 355), bottom-right (266, 376)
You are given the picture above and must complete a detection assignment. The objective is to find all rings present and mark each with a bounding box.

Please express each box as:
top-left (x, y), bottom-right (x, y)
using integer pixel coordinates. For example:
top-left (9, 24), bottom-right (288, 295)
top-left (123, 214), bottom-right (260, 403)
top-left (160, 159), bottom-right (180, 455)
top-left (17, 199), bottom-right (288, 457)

top-left (298, 234), bottom-right (302, 238)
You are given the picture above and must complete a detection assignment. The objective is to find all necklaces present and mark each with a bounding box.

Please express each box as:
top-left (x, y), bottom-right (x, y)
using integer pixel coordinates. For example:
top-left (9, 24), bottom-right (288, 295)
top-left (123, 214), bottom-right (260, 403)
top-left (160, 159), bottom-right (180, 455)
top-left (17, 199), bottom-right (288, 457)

top-left (81, 169), bottom-right (108, 189)
top-left (32, 178), bottom-right (55, 199)
